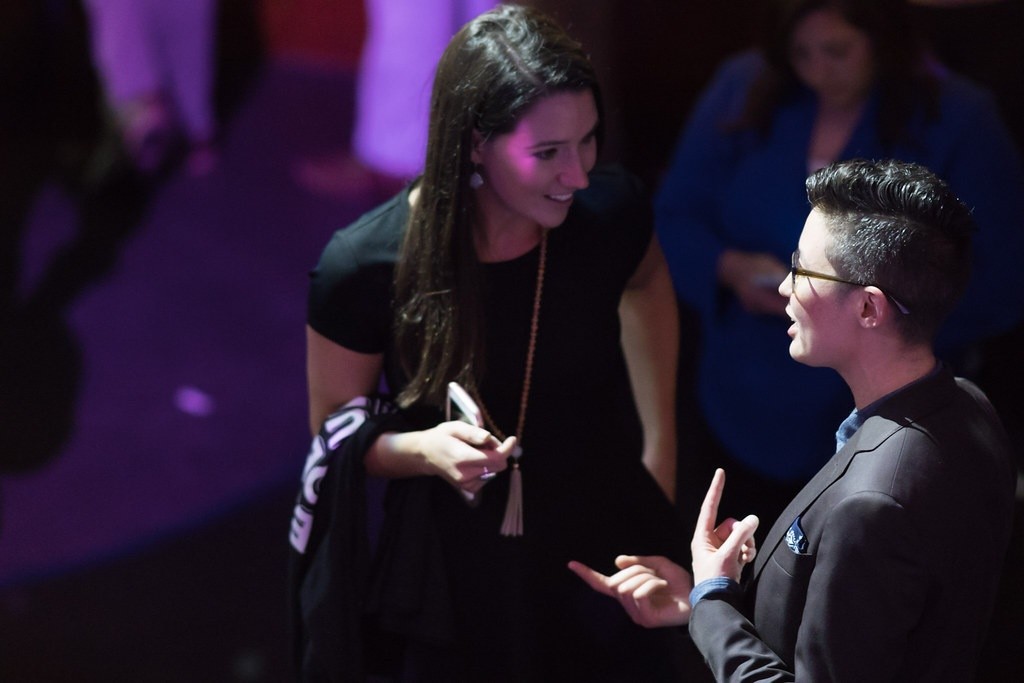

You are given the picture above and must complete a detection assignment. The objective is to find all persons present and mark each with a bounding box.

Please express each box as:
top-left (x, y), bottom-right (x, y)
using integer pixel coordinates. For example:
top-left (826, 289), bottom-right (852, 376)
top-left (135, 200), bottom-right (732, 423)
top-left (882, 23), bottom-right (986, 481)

top-left (88, 0), bottom-right (1024, 683)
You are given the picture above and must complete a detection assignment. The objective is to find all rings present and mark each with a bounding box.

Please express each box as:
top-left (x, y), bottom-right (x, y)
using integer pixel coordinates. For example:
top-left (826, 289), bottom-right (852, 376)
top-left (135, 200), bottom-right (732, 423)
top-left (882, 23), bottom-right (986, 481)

top-left (479, 466), bottom-right (495, 479)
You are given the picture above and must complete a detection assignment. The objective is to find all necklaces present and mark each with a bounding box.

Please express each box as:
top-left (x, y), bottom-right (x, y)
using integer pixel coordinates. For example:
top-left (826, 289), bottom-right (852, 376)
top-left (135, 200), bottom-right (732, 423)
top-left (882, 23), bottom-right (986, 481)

top-left (466, 226), bottom-right (550, 536)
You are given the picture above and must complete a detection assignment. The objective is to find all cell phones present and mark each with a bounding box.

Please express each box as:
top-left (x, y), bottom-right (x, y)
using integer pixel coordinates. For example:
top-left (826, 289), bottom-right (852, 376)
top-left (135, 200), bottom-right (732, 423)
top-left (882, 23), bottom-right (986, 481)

top-left (446, 382), bottom-right (486, 429)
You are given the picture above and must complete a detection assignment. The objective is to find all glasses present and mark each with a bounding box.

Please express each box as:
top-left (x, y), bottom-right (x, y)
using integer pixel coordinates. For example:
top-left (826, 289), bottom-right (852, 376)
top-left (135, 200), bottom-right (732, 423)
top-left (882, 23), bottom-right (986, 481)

top-left (790, 252), bottom-right (910, 316)
